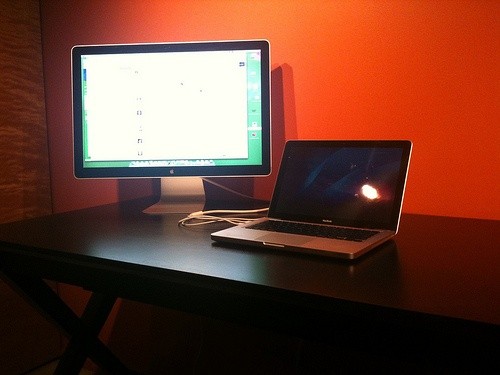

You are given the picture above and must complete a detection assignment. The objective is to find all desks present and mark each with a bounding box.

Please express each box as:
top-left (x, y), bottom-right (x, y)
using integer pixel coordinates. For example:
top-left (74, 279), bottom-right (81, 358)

top-left (0, 195), bottom-right (500, 375)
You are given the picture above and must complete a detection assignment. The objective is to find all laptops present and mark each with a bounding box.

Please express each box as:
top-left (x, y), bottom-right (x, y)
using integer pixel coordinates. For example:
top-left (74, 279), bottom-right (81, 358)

top-left (209, 138), bottom-right (414, 261)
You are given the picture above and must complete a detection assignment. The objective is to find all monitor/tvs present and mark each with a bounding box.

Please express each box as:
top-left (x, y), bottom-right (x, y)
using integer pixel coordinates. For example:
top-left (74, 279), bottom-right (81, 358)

top-left (70, 38), bottom-right (273, 178)
